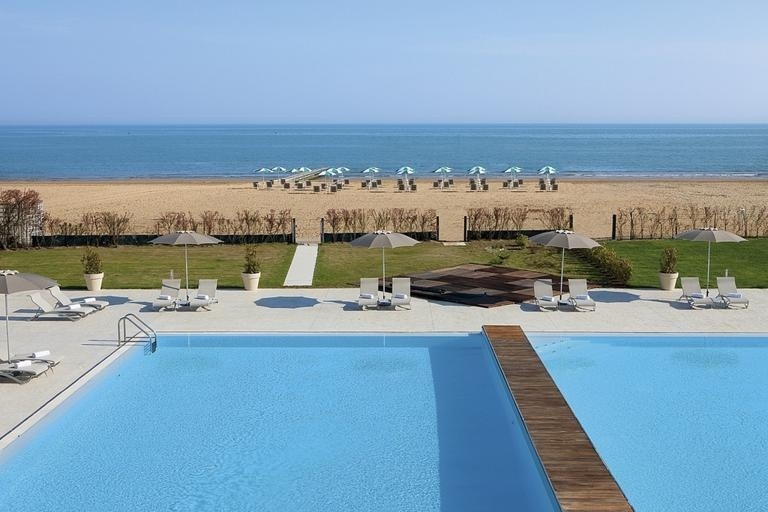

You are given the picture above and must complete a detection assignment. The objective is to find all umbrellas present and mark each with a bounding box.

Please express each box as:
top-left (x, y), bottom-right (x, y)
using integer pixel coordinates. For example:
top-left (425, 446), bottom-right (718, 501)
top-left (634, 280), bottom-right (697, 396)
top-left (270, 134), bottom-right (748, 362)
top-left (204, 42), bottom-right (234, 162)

top-left (672, 227), bottom-right (751, 295)
top-left (146, 229), bottom-right (226, 300)
top-left (528, 229), bottom-right (604, 300)
top-left (0, 269), bottom-right (58, 363)
top-left (347, 229), bottom-right (423, 299)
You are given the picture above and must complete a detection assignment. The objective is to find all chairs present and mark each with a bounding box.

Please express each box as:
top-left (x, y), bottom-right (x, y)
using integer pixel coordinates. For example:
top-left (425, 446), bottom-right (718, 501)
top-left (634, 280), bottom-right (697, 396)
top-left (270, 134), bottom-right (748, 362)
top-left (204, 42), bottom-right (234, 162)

top-left (429, 178), bottom-right (457, 193)
top-left (465, 178), bottom-right (489, 193)
top-left (251, 178), bottom-right (350, 194)
top-left (677, 274), bottom-right (751, 311)
top-left (531, 276), bottom-right (596, 313)
top-left (394, 178), bottom-right (418, 193)
top-left (534, 177), bottom-right (560, 193)
top-left (358, 277), bottom-right (412, 311)
top-left (360, 178), bottom-right (383, 191)
top-left (0, 277), bottom-right (219, 385)
top-left (498, 179), bottom-right (528, 191)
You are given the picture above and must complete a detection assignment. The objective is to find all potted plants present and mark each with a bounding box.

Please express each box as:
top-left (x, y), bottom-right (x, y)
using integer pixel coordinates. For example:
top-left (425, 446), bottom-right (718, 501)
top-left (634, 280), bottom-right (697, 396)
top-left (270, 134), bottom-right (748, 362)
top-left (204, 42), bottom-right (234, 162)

top-left (241, 243), bottom-right (262, 292)
top-left (84, 246), bottom-right (105, 292)
top-left (657, 245), bottom-right (681, 290)
